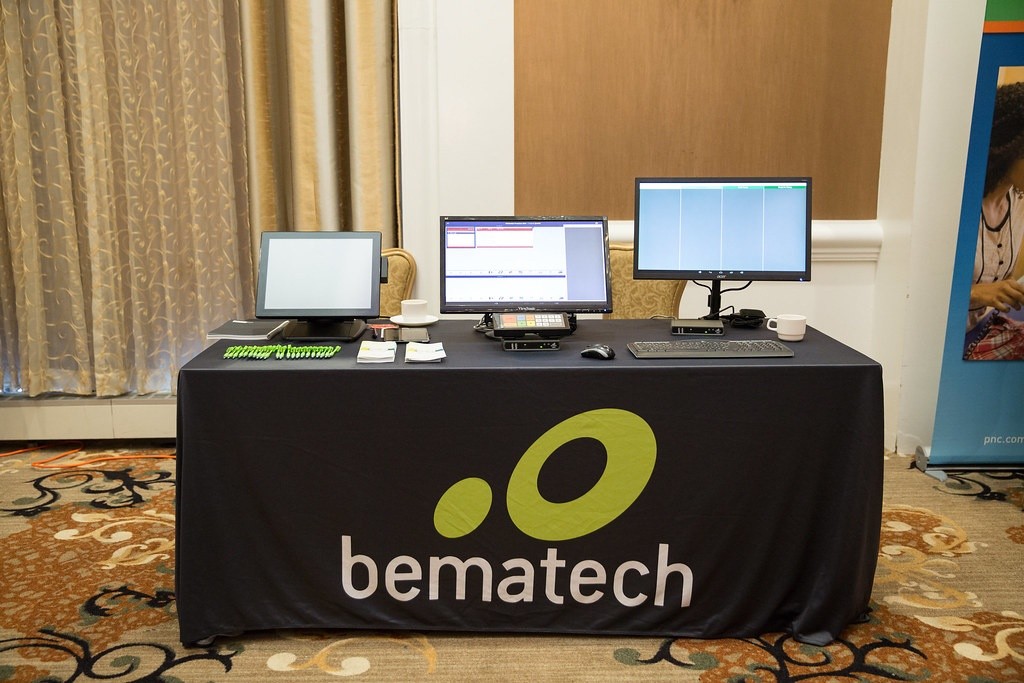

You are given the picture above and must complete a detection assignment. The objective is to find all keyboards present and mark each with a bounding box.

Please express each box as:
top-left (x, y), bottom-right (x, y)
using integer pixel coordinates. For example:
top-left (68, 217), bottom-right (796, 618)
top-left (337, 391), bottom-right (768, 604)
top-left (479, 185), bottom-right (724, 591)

top-left (626, 339), bottom-right (794, 359)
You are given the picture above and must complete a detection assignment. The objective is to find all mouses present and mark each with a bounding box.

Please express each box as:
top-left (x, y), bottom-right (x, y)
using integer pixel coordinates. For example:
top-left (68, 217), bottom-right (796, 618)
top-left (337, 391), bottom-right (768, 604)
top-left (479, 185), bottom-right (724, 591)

top-left (581, 343), bottom-right (615, 360)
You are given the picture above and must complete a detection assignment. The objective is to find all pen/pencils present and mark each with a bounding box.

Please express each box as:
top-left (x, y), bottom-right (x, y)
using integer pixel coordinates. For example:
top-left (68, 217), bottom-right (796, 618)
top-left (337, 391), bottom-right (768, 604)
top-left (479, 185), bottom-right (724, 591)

top-left (373, 327), bottom-right (378, 337)
top-left (222, 344), bottom-right (342, 360)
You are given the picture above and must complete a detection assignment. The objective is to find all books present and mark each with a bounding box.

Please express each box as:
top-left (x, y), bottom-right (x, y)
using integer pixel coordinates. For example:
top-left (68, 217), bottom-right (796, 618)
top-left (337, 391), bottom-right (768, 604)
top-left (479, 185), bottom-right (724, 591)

top-left (205, 320), bottom-right (289, 340)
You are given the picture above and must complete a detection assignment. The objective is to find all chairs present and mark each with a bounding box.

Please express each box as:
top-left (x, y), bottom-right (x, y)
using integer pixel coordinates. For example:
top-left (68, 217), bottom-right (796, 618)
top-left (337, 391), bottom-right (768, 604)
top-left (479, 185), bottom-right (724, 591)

top-left (602, 241), bottom-right (688, 320)
top-left (380, 248), bottom-right (417, 318)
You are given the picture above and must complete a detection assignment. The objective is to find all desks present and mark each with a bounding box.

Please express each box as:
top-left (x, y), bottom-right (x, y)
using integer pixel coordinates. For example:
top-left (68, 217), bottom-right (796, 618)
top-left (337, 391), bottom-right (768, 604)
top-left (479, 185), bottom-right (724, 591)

top-left (174, 319), bottom-right (884, 650)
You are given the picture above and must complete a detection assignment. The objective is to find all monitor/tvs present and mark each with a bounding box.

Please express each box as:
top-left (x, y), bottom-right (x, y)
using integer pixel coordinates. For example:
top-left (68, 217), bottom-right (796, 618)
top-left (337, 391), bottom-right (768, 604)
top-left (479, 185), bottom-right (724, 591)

top-left (255, 230), bottom-right (382, 341)
top-left (633, 176), bottom-right (811, 321)
top-left (439, 215), bottom-right (613, 338)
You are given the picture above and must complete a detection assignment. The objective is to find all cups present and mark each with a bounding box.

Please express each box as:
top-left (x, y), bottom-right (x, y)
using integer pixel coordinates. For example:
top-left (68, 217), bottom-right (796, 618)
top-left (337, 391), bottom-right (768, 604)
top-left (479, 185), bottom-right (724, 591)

top-left (400, 300), bottom-right (428, 322)
top-left (767, 315), bottom-right (806, 341)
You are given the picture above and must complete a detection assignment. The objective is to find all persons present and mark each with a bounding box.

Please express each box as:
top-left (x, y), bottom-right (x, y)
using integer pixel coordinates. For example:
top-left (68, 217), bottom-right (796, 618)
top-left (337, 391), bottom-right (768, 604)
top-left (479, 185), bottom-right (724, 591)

top-left (964, 82), bottom-right (1024, 360)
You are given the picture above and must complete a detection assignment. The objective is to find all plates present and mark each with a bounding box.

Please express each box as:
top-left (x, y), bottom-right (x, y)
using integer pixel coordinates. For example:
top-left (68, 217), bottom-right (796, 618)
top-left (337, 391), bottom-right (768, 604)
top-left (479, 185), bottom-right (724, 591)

top-left (390, 315), bottom-right (439, 326)
